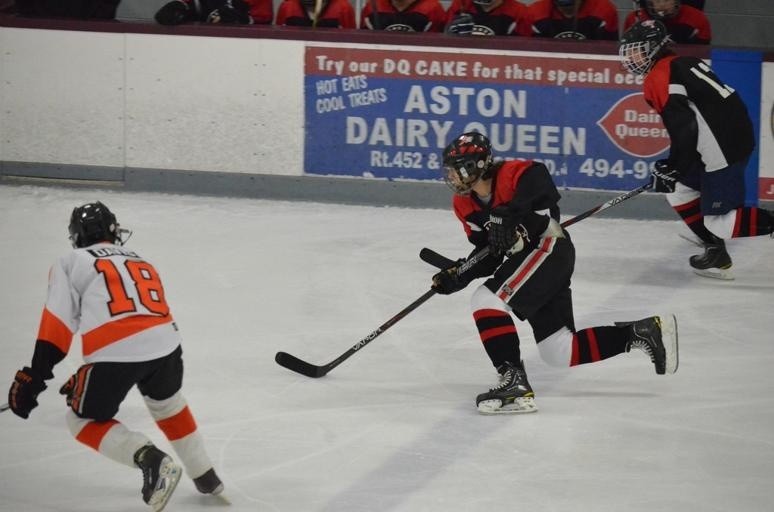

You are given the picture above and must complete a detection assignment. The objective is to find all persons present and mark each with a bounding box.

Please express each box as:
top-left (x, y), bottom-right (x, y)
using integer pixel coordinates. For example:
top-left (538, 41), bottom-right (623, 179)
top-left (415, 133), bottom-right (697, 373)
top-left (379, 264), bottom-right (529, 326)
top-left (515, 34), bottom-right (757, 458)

top-left (154, 0), bottom-right (722, 46)
top-left (430, 132), bottom-right (667, 407)
top-left (8, 200), bottom-right (224, 506)
top-left (619, 19), bottom-right (774, 270)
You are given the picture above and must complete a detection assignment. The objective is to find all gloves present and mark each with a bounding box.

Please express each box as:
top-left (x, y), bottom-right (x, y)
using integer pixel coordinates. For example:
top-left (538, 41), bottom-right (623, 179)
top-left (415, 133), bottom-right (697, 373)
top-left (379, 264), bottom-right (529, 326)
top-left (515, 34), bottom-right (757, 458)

top-left (430, 266), bottom-right (469, 294)
top-left (643, 159), bottom-right (692, 194)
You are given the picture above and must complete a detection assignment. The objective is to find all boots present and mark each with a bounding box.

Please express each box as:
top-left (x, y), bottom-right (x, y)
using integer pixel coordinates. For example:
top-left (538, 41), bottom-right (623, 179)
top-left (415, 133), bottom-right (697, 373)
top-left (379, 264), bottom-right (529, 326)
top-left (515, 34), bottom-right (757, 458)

top-left (475, 359), bottom-right (534, 406)
top-left (191, 467), bottom-right (221, 494)
top-left (689, 238), bottom-right (733, 270)
top-left (614, 316), bottom-right (667, 375)
top-left (134, 443), bottom-right (173, 504)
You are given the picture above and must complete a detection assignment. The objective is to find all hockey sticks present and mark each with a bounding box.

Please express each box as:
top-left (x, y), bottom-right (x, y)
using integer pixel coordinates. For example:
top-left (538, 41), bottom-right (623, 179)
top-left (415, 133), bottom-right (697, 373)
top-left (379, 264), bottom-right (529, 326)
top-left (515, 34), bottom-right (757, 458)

top-left (419, 183), bottom-right (653, 269)
top-left (276, 233), bottom-right (520, 378)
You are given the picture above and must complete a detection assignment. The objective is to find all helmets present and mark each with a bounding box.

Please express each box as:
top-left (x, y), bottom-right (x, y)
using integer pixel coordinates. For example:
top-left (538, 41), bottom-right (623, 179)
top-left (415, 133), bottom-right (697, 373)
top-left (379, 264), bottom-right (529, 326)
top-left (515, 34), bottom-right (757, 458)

top-left (618, 18), bottom-right (671, 76)
top-left (66, 201), bottom-right (132, 249)
top-left (439, 133), bottom-right (493, 197)
